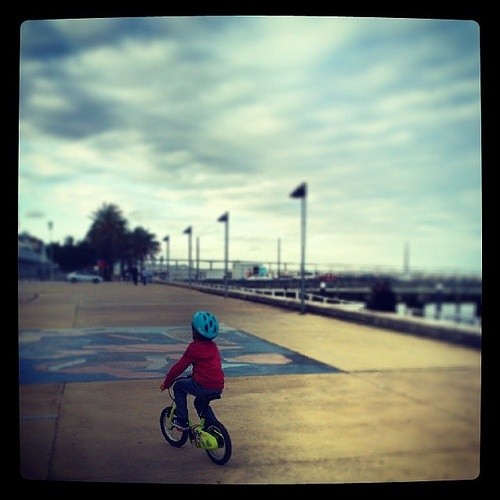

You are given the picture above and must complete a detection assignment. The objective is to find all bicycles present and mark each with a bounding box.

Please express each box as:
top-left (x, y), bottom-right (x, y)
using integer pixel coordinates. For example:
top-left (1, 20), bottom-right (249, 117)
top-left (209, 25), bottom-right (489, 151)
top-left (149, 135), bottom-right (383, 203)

top-left (160, 376), bottom-right (232, 465)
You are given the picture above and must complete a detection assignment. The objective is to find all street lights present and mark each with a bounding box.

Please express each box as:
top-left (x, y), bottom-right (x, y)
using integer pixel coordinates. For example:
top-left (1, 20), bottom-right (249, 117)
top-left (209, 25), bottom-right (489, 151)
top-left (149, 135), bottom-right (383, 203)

top-left (162, 235), bottom-right (170, 285)
top-left (217, 211), bottom-right (229, 299)
top-left (184, 226), bottom-right (191, 290)
top-left (288, 183), bottom-right (307, 315)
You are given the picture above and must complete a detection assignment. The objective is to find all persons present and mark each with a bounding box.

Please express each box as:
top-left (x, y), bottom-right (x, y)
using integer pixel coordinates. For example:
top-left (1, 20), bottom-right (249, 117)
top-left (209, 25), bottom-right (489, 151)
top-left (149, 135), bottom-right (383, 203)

top-left (142, 266), bottom-right (148, 286)
top-left (132, 265), bottom-right (139, 285)
top-left (161, 311), bottom-right (224, 432)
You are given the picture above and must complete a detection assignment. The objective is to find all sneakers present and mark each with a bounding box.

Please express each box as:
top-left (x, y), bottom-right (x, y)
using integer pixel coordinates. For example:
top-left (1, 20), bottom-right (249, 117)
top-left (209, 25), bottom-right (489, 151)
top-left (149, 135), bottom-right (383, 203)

top-left (170, 417), bottom-right (190, 430)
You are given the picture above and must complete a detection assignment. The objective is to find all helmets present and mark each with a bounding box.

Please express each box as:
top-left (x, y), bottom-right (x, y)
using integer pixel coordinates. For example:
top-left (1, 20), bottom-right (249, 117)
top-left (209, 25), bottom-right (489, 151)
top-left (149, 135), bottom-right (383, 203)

top-left (193, 310), bottom-right (219, 339)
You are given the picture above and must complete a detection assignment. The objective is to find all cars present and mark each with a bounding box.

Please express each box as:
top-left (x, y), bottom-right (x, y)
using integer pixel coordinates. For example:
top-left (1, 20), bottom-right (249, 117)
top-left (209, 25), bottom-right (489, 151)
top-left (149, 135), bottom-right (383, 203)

top-left (66, 270), bottom-right (103, 284)
